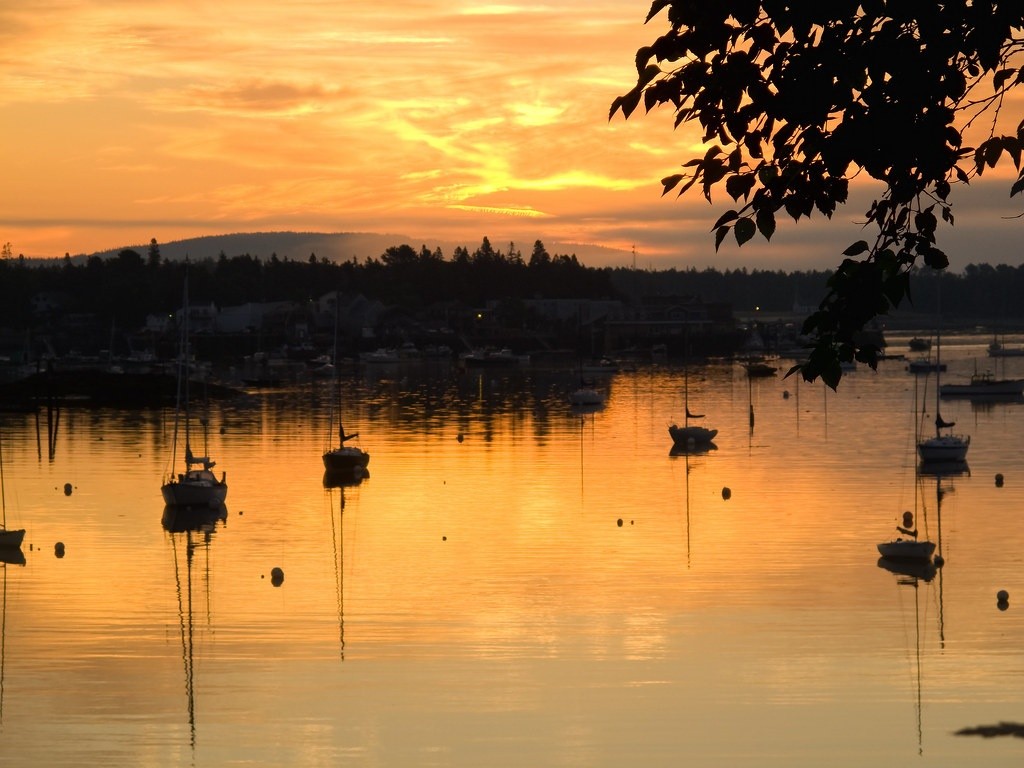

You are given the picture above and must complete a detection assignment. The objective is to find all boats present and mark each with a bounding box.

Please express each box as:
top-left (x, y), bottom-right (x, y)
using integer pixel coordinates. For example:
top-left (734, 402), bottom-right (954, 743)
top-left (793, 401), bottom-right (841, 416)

top-left (939, 355), bottom-right (1024, 397)
top-left (975, 325), bottom-right (986, 334)
top-left (600, 359), bottom-right (622, 372)
top-left (373, 342), bottom-right (531, 364)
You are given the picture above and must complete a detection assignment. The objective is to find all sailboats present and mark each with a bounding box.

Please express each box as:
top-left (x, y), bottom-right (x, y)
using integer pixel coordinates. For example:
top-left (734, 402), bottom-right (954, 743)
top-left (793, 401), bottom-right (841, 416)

top-left (739, 319), bottom-right (777, 375)
top-left (988, 295), bottom-right (1024, 357)
top-left (570, 307), bottom-right (602, 404)
top-left (160, 255), bottom-right (229, 507)
top-left (322, 286), bottom-right (370, 474)
top-left (877, 368), bottom-right (936, 558)
top-left (0, 452), bottom-right (25, 549)
top-left (910, 316), bottom-right (945, 372)
top-left (914, 284), bottom-right (972, 463)
top-left (668, 311), bottom-right (719, 445)
